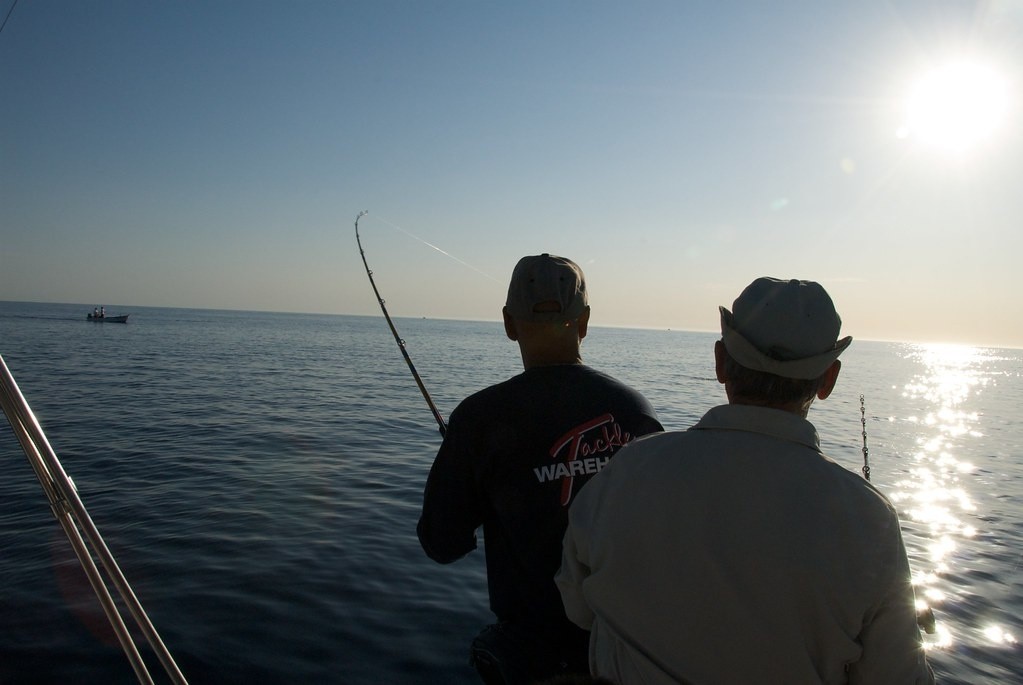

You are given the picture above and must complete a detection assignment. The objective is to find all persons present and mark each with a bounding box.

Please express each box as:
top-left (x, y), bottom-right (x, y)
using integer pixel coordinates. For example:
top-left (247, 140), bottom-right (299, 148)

top-left (414, 254), bottom-right (663, 684)
top-left (95, 307), bottom-right (99, 317)
top-left (553, 274), bottom-right (940, 685)
top-left (100, 307), bottom-right (105, 318)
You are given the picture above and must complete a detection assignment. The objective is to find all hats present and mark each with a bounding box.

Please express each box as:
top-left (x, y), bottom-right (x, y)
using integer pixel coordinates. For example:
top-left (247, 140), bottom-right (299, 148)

top-left (505, 252), bottom-right (588, 321)
top-left (719, 277), bottom-right (853, 381)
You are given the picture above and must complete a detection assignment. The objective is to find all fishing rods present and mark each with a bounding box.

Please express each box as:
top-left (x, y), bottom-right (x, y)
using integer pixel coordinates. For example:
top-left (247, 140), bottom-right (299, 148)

top-left (354, 211), bottom-right (449, 439)
top-left (860, 393), bottom-right (871, 480)
top-left (0, 354), bottom-right (189, 685)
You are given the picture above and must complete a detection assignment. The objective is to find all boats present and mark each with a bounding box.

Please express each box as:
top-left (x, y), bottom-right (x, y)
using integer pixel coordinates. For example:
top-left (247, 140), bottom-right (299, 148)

top-left (86, 314), bottom-right (131, 323)
